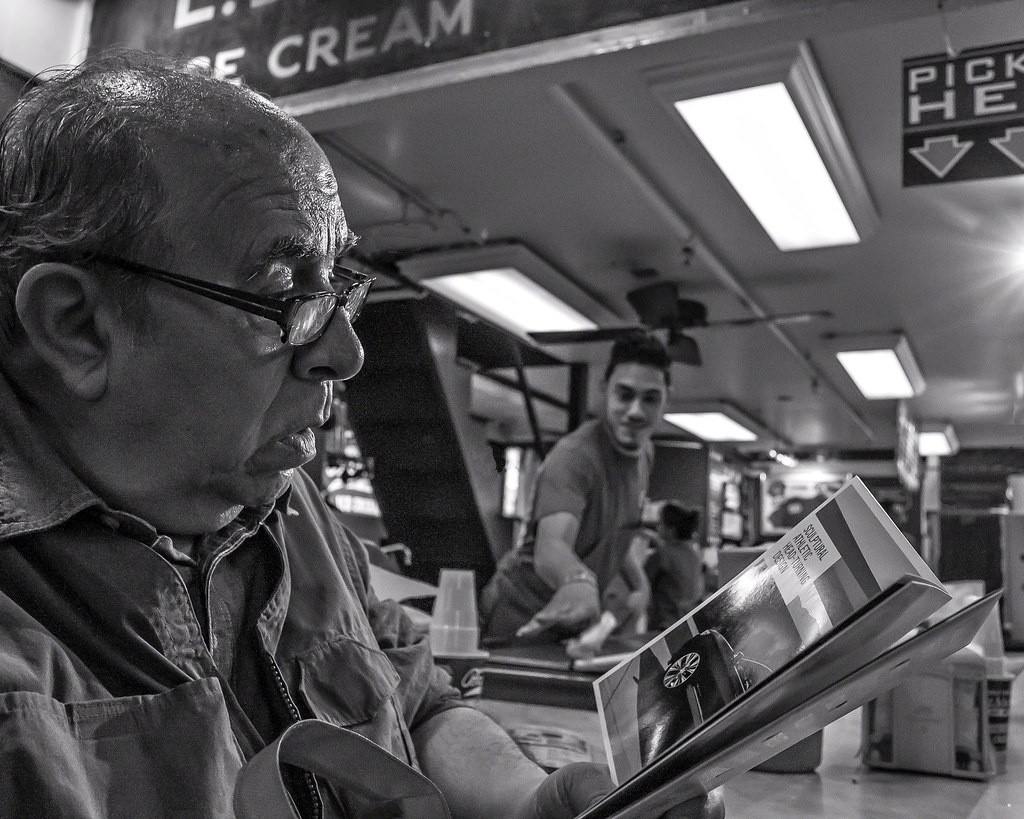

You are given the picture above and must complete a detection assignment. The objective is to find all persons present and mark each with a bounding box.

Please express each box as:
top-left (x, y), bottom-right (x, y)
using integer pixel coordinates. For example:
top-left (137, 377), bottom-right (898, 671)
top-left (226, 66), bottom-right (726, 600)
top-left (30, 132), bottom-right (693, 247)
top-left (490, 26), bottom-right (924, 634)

top-left (478, 331), bottom-right (671, 646)
top-left (641, 502), bottom-right (704, 632)
top-left (0, 56), bottom-right (725, 819)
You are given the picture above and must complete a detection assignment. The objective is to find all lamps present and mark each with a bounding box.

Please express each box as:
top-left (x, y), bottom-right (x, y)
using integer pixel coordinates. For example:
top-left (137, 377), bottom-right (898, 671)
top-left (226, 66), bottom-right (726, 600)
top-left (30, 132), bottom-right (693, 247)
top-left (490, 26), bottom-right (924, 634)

top-left (662, 400), bottom-right (772, 449)
top-left (642, 36), bottom-right (886, 265)
top-left (397, 236), bottom-right (622, 343)
top-left (823, 332), bottom-right (927, 402)
top-left (914, 421), bottom-right (959, 456)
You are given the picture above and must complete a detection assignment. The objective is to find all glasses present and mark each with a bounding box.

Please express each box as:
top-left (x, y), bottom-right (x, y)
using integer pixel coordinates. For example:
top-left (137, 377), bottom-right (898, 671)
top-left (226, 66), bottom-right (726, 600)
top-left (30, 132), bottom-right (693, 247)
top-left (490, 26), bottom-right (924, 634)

top-left (40, 252), bottom-right (377, 349)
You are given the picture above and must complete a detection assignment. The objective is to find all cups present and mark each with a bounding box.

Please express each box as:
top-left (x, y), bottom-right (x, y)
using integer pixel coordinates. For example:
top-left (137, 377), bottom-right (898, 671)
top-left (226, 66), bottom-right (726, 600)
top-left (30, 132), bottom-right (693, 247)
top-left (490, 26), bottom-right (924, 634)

top-left (429, 568), bottom-right (490, 658)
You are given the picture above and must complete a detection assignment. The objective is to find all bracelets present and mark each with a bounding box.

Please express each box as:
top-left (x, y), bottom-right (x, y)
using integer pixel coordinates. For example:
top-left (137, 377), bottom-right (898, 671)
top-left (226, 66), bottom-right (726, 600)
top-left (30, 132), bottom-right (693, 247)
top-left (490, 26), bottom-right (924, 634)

top-left (562, 569), bottom-right (597, 585)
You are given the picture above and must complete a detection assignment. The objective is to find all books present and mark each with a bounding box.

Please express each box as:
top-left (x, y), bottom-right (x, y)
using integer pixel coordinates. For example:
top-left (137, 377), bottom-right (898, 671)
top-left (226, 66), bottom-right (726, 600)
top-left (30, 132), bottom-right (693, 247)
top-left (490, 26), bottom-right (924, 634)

top-left (592, 476), bottom-right (952, 788)
